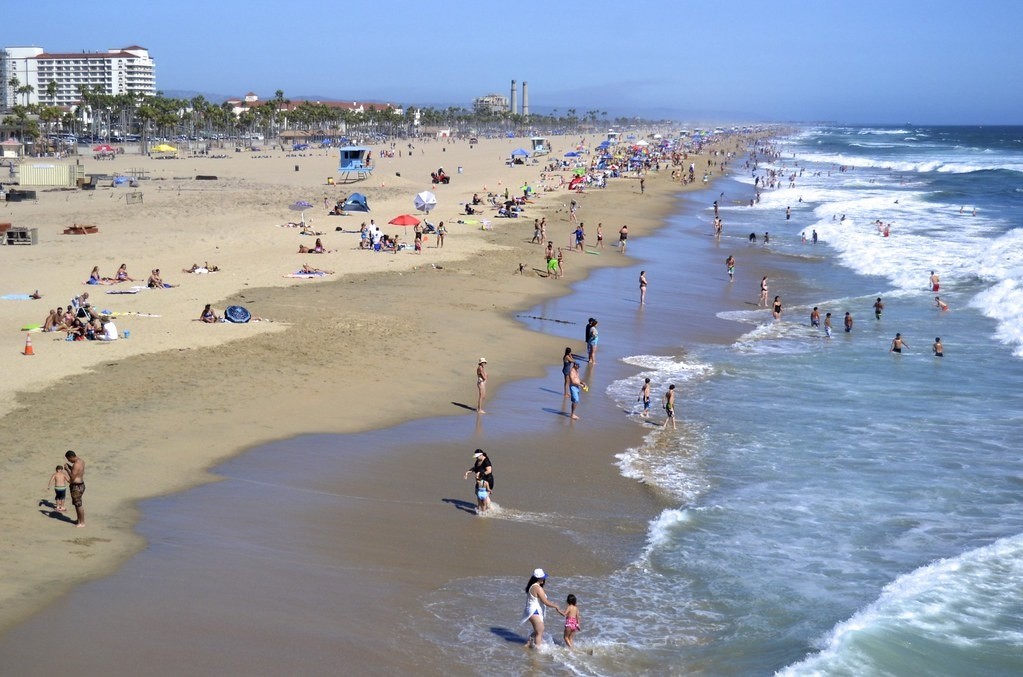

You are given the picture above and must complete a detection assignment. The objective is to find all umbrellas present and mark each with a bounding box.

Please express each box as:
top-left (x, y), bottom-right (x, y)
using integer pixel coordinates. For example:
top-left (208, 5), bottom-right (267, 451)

top-left (599, 155), bottom-right (614, 166)
top-left (631, 157), bottom-right (642, 161)
top-left (520, 187), bottom-right (535, 194)
top-left (225, 306), bottom-right (251, 324)
top-left (601, 140), bottom-right (611, 145)
top-left (414, 191), bottom-right (438, 212)
top-left (598, 144), bottom-right (608, 149)
top-left (288, 200), bottom-right (314, 223)
top-left (568, 175), bottom-right (584, 190)
top-left (388, 215), bottom-right (422, 244)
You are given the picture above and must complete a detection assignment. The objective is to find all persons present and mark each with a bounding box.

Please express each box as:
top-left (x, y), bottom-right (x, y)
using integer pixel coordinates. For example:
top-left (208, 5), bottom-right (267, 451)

top-left (465, 449), bottom-right (495, 514)
top-left (43, 127), bottom-right (977, 364)
top-left (476, 358), bottom-right (488, 414)
top-left (63, 450), bottom-right (87, 528)
top-left (475, 472), bottom-right (492, 516)
top-left (47, 466), bottom-right (69, 511)
top-left (561, 347), bottom-right (576, 398)
top-left (520, 567), bottom-right (558, 650)
top-left (637, 378), bottom-right (652, 416)
top-left (569, 360), bottom-right (586, 420)
top-left (663, 385), bottom-right (679, 430)
top-left (557, 594), bottom-right (581, 647)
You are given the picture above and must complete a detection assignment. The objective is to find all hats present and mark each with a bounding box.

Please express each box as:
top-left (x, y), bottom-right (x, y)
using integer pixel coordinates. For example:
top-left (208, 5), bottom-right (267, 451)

top-left (533, 568), bottom-right (548, 578)
top-left (588, 318), bottom-right (595, 323)
top-left (574, 361), bottom-right (581, 366)
top-left (472, 452), bottom-right (483, 458)
top-left (478, 358), bottom-right (487, 364)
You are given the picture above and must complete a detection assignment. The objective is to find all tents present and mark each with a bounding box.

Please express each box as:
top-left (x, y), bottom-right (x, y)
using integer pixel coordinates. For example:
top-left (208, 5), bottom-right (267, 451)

top-left (149, 143), bottom-right (178, 159)
top-left (343, 192), bottom-right (369, 211)
top-left (680, 130), bottom-right (690, 137)
top-left (564, 152), bottom-right (579, 162)
top-left (511, 148), bottom-right (530, 161)
top-left (636, 140), bottom-right (649, 147)
top-left (653, 134), bottom-right (662, 140)
top-left (93, 144), bottom-right (117, 161)
